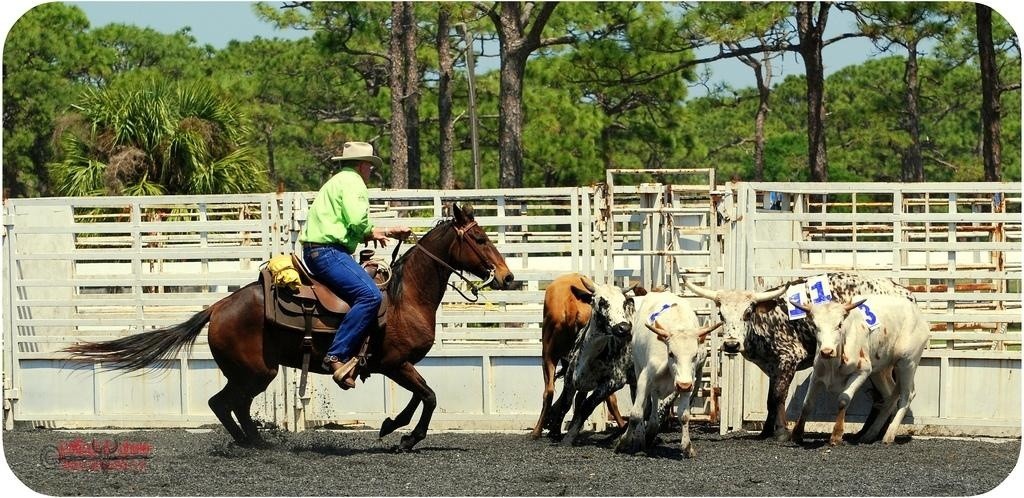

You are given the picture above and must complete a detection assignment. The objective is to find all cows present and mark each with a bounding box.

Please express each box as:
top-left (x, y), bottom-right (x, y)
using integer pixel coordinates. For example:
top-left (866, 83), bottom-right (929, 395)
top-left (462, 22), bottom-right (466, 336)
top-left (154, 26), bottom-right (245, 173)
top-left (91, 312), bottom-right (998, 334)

top-left (526, 273), bottom-right (933, 460)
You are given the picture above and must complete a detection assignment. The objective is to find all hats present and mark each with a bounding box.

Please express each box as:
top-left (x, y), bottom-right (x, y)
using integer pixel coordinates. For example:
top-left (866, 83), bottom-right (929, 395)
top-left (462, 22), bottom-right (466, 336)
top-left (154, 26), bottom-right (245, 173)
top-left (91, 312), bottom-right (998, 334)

top-left (330, 141), bottom-right (383, 169)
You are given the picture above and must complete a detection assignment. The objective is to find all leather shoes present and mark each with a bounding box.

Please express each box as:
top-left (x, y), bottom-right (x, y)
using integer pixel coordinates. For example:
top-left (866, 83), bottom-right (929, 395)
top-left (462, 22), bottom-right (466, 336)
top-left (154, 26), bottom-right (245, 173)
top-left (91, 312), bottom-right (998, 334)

top-left (321, 355), bottom-right (356, 388)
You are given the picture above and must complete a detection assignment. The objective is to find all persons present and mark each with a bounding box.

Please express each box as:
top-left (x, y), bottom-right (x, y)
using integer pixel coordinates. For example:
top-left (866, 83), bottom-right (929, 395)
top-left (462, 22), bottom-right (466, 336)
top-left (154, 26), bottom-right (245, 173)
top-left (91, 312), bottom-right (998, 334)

top-left (302, 142), bottom-right (412, 387)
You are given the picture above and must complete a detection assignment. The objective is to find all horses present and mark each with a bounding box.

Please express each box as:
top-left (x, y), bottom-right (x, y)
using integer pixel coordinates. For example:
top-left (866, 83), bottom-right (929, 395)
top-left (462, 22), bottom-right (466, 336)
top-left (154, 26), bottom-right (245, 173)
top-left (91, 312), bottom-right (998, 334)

top-left (58, 202), bottom-right (514, 453)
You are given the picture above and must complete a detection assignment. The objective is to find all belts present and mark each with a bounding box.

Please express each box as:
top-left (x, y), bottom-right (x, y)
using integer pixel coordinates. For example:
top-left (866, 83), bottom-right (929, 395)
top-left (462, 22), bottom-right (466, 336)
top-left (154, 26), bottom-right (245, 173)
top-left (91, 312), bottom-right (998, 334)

top-left (301, 242), bottom-right (325, 250)
